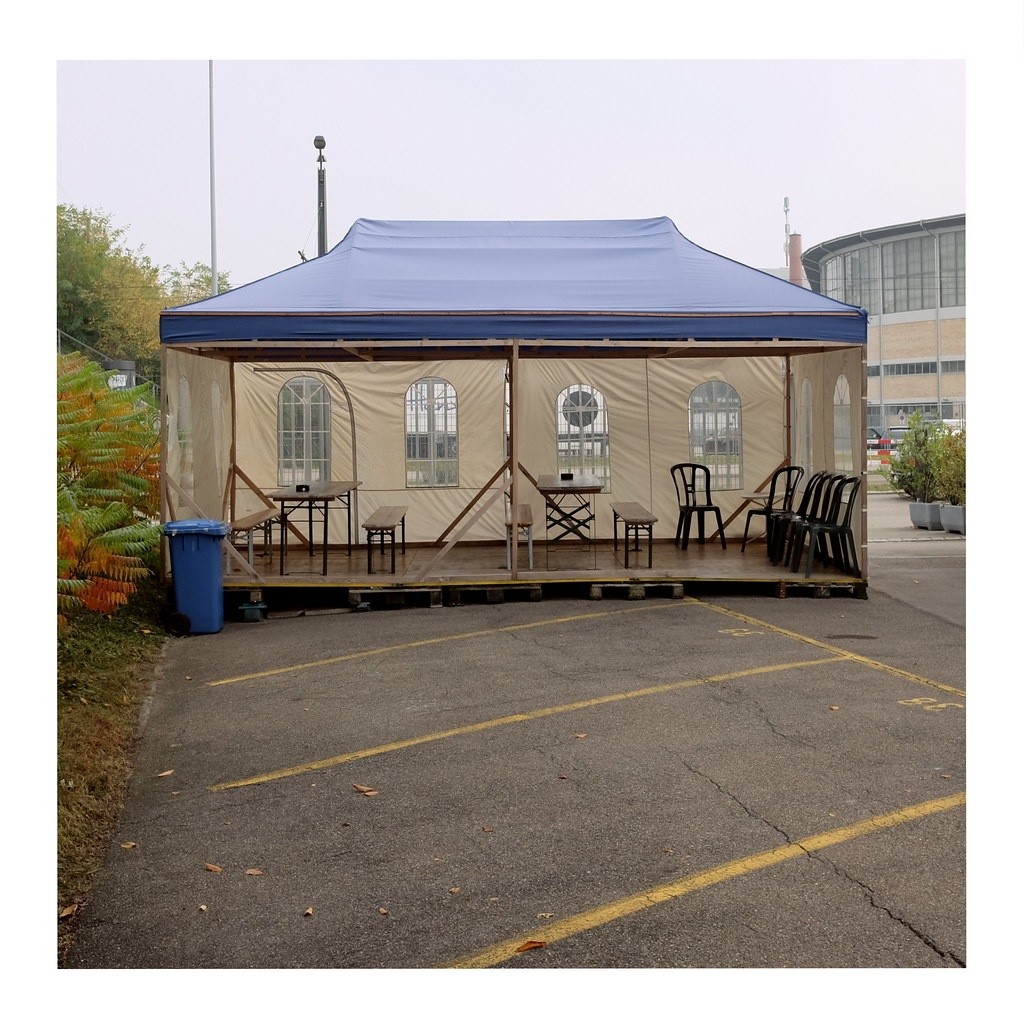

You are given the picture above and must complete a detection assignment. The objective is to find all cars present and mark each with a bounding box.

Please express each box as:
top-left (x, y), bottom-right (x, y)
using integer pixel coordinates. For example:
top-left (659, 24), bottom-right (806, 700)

top-left (927, 426), bottom-right (948, 442)
top-left (702, 425), bottom-right (739, 456)
top-left (950, 427), bottom-right (963, 436)
top-left (866, 427), bottom-right (883, 449)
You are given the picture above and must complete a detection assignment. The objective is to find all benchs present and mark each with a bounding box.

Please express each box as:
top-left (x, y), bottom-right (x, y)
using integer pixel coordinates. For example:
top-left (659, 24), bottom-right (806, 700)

top-left (505, 503), bottom-right (533, 570)
top-left (226, 508), bottom-right (291, 575)
top-left (361, 505), bottom-right (409, 575)
top-left (607, 502), bottom-right (658, 570)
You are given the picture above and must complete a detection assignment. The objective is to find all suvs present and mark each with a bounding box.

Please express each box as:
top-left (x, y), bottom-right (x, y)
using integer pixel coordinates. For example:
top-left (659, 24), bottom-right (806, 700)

top-left (879, 426), bottom-right (912, 450)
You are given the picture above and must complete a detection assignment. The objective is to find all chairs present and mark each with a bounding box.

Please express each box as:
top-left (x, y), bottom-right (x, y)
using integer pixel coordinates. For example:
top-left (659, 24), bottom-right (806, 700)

top-left (784, 475), bottom-right (846, 572)
top-left (792, 477), bottom-right (862, 579)
top-left (741, 466), bottom-right (805, 556)
top-left (669, 463), bottom-right (727, 552)
top-left (770, 469), bottom-right (827, 562)
top-left (772, 473), bottom-right (834, 567)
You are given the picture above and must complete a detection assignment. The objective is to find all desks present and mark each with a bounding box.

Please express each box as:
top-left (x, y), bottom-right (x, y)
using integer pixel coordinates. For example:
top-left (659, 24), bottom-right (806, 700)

top-left (742, 492), bottom-right (791, 544)
top-left (536, 474), bottom-right (605, 571)
top-left (264, 481), bottom-right (363, 576)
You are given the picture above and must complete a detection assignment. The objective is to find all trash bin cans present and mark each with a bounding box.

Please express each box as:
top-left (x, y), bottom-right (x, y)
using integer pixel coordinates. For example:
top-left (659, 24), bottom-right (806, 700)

top-left (163, 519), bottom-right (234, 636)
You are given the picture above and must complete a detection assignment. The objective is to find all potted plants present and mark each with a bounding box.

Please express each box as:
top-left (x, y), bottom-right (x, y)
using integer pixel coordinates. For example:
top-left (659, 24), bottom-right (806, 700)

top-left (877, 411), bottom-right (955, 531)
top-left (930, 425), bottom-right (966, 535)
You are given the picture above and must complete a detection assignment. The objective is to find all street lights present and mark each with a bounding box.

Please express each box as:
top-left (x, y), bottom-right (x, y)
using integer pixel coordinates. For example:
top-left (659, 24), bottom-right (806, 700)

top-left (314, 136), bottom-right (328, 257)
